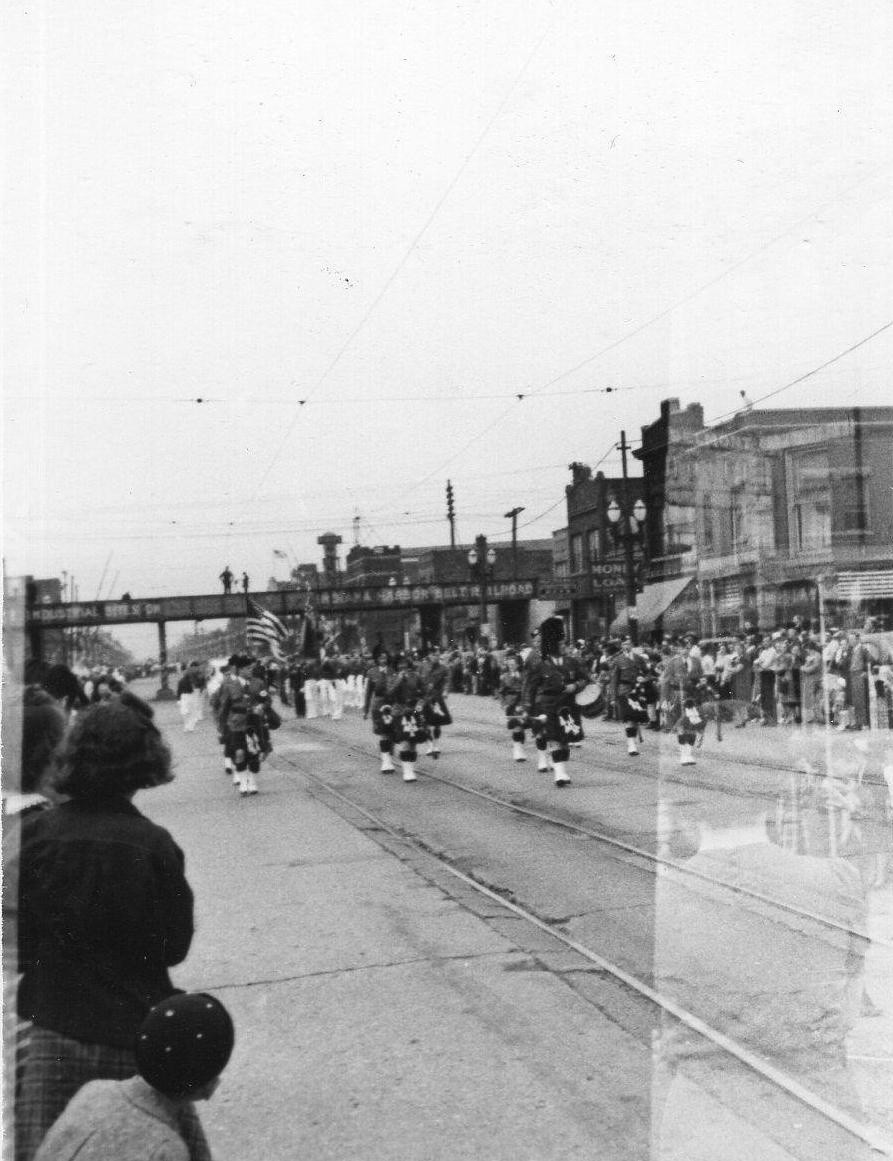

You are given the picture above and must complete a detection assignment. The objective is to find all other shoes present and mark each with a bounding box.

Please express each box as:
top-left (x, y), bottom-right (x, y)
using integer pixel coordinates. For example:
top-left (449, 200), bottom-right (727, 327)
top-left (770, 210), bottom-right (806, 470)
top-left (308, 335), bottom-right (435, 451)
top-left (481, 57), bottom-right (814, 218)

top-left (703, 715), bottom-right (872, 732)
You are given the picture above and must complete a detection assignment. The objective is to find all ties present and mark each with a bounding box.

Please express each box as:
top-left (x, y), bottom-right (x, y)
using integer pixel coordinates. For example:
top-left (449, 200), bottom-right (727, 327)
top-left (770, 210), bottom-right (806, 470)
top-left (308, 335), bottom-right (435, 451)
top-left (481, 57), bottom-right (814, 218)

top-left (556, 658), bottom-right (560, 669)
top-left (244, 683), bottom-right (249, 693)
top-left (685, 660), bottom-right (688, 670)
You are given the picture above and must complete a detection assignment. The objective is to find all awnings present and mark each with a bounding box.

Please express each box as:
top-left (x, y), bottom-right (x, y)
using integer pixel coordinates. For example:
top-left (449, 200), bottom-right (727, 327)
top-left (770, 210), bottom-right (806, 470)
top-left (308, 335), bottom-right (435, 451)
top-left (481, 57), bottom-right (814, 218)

top-left (609, 576), bottom-right (694, 635)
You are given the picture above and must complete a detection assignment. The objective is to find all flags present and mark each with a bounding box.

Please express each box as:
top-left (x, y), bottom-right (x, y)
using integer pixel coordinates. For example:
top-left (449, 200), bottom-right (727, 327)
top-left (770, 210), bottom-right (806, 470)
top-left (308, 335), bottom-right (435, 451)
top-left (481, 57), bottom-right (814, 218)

top-left (245, 598), bottom-right (287, 650)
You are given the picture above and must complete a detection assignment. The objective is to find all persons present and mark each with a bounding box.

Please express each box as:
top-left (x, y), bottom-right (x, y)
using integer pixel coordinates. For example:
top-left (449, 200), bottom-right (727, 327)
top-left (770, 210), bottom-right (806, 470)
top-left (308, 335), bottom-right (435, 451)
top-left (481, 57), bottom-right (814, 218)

top-left (34, 992), bottom-right (234, 1161)
top-left (122, 591), bottom-right (131, 600)
top-left (16, 649), bottom-right (211, 1161)
top-left (43, 593), bottom-right (52, 604)
top-left (205, 617), bottom-right (893, 795)
top-left (388, 576), bottom-right (412, 587)
top-left (219, 566), bottom-right (249, 594)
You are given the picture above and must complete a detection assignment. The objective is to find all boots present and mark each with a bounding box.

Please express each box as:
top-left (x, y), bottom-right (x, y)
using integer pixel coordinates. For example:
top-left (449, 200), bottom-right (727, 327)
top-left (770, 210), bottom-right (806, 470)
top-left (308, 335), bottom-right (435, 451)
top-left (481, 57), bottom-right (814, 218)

top-left (224, 757), bottom-right (241, 786)
top-left (536, 749), bottom-right (554, 773)
top-left (513, 741), bottom-right (528, 762)
top-left (400, 759), bottom-right (418, 783)
top-left (627, 738), bottom-right (640, 755)
top-left (239, 770), bottom-right (260, 796)
top-left (426, 738), bottom-right (441, 758)
top-left (379, 751), bottom-right (397, 773)
top-left (555, 761), bottom-right (572, 786)
top-left (679, 744), bottom-right (696, 766)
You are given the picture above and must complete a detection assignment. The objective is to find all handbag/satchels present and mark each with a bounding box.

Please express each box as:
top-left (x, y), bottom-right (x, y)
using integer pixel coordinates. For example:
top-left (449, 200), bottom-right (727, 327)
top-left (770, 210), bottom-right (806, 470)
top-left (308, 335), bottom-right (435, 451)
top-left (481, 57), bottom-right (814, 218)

top-left (776, 669), bottom-right (789, 696)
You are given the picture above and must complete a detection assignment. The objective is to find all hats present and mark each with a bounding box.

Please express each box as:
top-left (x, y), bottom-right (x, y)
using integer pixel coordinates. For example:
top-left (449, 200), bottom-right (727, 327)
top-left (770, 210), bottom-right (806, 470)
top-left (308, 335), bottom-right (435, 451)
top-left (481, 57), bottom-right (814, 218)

top-left (221, 654), bottom-right (266, 673)
top-left (621, 634), bottom-right (632, 643)
top-left (189, 661), bottom-right (200, 668)
top-left (372, 645), bottom-right (439, 667)
top-left (531, 617), bottom-right (568, 642)
top-left (134, 992), bottom-right (234, 1097)
top-left (504, 648), bottom-right (517, 655)
top-left (833, 630), bottom-right (845, 639)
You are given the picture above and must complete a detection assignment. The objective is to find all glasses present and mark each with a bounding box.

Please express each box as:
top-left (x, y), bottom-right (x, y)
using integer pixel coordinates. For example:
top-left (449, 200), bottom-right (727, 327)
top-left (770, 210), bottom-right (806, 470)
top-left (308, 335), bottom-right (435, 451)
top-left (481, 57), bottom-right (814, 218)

top-left (95, 692), bottom-right (111, 702)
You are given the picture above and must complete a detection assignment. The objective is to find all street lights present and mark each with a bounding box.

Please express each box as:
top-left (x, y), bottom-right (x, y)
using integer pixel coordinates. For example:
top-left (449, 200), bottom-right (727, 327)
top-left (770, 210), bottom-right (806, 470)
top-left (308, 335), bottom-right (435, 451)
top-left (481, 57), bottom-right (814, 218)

top-left (467, 549), bottom-right (497, 694)
top-left (608, 500), bottom-right (648, 647)
